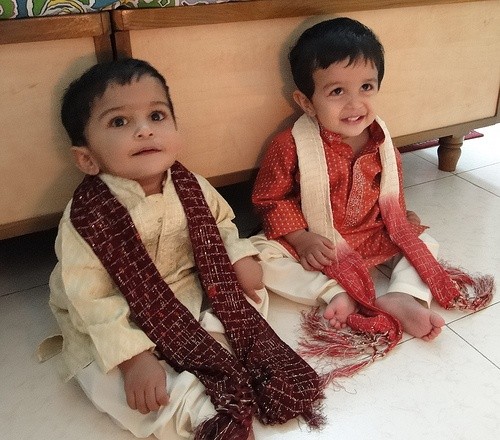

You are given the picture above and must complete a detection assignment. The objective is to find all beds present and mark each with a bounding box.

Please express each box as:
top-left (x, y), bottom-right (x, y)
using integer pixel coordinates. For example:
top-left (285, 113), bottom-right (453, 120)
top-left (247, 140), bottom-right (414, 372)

top-left (1, 0), bottom-right (500, 237)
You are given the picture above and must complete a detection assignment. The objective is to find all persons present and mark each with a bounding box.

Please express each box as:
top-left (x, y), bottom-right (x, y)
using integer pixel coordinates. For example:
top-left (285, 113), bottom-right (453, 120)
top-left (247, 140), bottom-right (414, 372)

top-left (249, 17), bottom-right (444, 342)
top-left (36, 58), bottom-right (270, 440)
